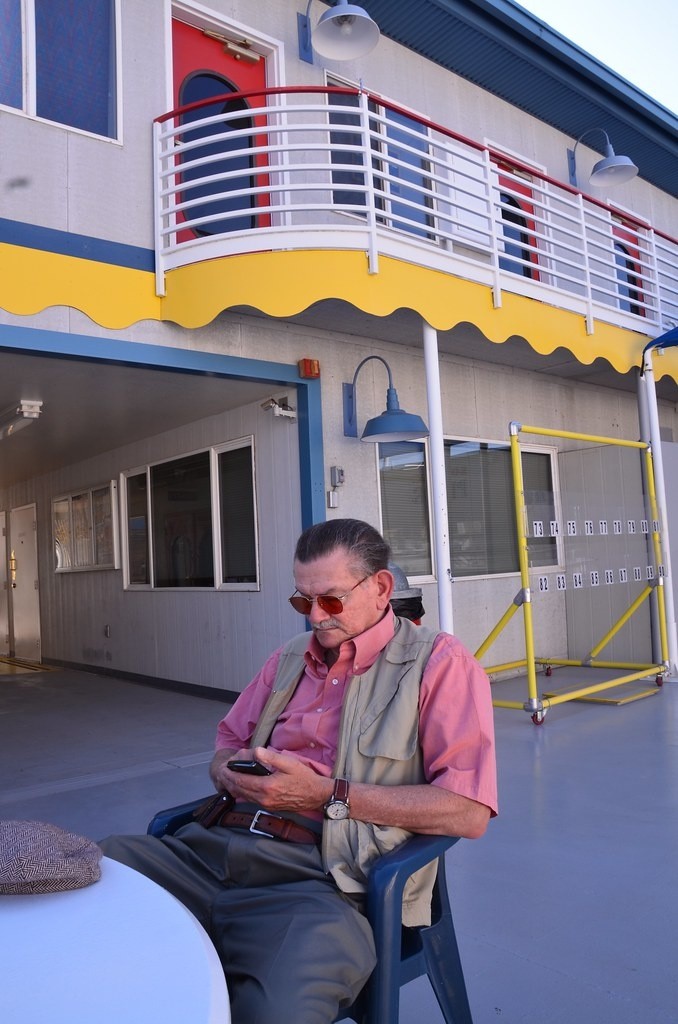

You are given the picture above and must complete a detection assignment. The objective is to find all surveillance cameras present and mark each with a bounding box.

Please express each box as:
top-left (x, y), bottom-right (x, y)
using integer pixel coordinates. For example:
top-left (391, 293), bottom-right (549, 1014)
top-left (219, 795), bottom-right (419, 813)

top-left (261, 399), bottom-right (276, 411)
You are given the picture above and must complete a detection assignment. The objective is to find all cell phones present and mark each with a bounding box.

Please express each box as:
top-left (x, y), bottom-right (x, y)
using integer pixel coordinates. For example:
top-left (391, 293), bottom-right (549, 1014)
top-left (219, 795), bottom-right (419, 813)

top-left (227, 760), bottom-right (273, 777)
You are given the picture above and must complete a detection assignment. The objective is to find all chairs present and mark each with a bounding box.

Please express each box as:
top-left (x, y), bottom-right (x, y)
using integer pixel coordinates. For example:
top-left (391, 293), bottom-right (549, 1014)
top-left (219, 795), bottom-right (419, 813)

top-left (147, 793), bottom-right (471, 1024)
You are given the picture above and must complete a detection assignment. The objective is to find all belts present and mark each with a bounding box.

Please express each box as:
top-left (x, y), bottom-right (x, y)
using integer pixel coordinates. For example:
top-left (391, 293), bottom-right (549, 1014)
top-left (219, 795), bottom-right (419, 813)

top-left (221, 811), bottom-right (323, 852)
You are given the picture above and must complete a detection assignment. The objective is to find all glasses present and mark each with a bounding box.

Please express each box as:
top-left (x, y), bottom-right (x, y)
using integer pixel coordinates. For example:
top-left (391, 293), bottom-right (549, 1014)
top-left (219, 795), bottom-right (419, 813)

top-left (288, 573), bottom-right (373, 615)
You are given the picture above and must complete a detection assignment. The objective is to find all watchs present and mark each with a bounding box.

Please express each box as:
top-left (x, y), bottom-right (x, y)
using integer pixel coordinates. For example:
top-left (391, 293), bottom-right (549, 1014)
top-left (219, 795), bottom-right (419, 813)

top-left (323, 777), bottom-right (350, 821)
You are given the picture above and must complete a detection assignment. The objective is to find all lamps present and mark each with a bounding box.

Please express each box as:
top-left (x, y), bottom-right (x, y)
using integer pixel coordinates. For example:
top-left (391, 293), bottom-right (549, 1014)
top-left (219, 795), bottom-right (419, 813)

top-left (343, 356), bottom-right (429, 442)
top-left (566, 128), bottom-right (639, 187)
top-left (297, 0), bottom-right (380, 65)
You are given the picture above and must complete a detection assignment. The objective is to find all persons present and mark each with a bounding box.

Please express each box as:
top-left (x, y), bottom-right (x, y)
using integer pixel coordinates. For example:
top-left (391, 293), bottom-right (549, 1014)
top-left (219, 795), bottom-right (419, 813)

top-left (96, 518), bottom-right (498, 1024)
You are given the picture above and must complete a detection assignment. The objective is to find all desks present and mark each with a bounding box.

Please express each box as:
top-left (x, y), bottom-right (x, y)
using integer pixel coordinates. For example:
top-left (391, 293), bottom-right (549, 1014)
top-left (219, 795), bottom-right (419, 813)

top-left (0, 819), bottom-right (232, 1024)
